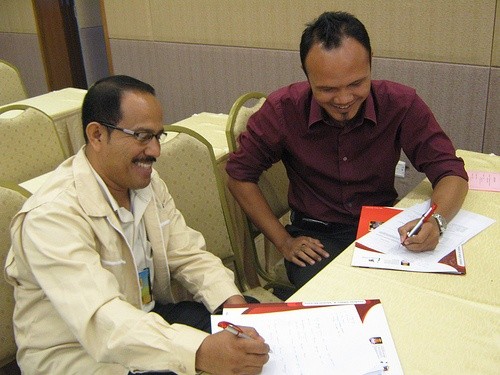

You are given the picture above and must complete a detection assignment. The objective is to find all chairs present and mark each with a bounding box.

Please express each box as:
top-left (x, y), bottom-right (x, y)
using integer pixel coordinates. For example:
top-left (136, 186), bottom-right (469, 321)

top-left (0, 59), bottom-right (29, 106)
top-left (0, 104), bottom-right (66, 197)
top-left (0, 177), bottom-right (32, 371)
top-left (153, 125), bottom-right (283, 304)
top-left (224, 91), bottom-right (297, 294)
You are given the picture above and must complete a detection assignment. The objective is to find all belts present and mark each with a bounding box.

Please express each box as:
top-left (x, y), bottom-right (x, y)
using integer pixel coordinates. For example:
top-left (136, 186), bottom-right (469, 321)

top-left (291, 211), bottom-right (345, 232)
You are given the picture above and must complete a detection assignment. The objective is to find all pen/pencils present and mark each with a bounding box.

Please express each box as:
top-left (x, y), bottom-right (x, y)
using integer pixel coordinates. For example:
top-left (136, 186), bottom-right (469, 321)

top-left (218, 322), bottom-right (272, 353)
top-left (400, 203), bottom-right (437, 248)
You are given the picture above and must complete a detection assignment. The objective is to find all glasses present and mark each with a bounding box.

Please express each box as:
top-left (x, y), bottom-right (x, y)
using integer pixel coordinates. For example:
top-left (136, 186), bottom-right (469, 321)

top-left (100, 122), bottom-right (167, 143)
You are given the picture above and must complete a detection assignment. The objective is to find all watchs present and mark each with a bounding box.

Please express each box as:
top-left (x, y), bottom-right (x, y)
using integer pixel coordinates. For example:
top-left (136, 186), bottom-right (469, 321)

top-left (422, 212), bottom-right (447, 236)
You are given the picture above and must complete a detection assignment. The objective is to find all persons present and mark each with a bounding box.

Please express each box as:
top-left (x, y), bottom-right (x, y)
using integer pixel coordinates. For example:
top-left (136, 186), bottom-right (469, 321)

top-left (225, 11), bottom-right (471, 291)
top-left (3, 74), bottom-right (269, 375)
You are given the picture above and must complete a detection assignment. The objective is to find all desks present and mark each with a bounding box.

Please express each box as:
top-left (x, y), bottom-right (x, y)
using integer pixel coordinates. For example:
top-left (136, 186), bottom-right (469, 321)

top-left (0, 82), bottom-right (500, 375)
